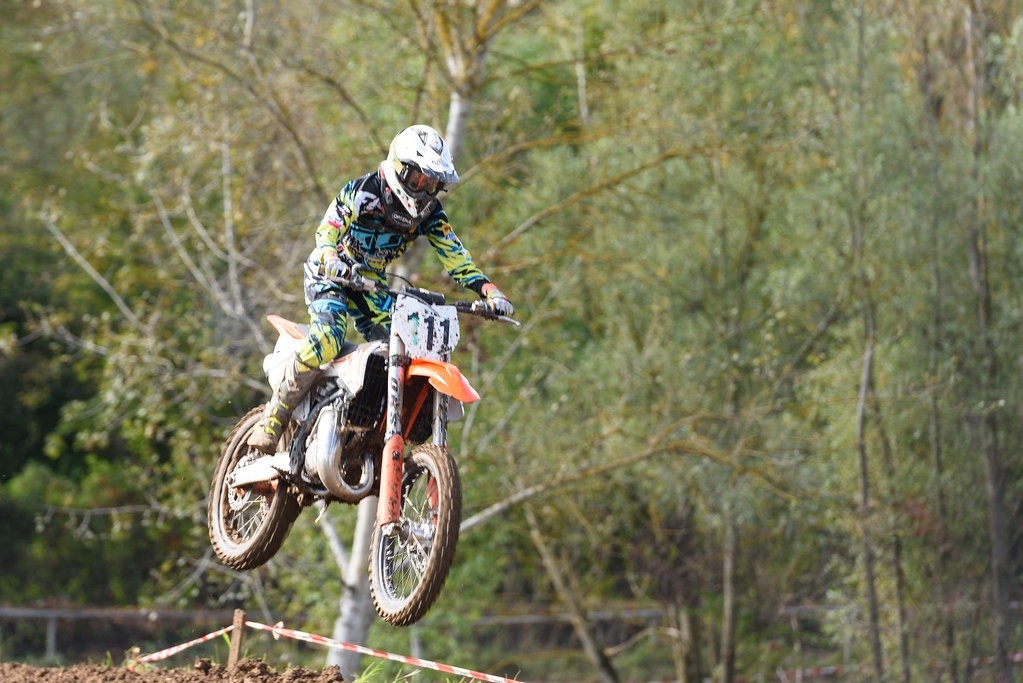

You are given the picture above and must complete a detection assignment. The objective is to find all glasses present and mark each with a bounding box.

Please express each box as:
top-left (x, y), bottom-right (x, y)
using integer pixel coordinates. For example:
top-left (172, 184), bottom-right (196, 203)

top-left (399, 164), bottom-right (442, 196)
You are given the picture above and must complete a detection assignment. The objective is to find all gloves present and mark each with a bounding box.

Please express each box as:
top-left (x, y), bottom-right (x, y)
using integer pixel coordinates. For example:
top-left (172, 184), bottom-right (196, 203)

top-left (481, 283), bottom-right (514, 321)
top-left (325, 258), bottom-right (352, 287)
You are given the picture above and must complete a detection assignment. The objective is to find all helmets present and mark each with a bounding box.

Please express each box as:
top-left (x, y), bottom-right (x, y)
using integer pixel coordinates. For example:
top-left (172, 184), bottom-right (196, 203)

top-left (381, 124), bottom-right (460, 219)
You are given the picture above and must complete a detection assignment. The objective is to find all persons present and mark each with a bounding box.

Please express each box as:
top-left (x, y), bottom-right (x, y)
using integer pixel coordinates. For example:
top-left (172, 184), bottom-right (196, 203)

top-left (249, 125), bottom-right (514, 452)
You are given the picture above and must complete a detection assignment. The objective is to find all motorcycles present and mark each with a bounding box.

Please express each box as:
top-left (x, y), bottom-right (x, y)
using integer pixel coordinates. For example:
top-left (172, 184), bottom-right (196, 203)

top-left (207, 262), bottom-right (519, 627)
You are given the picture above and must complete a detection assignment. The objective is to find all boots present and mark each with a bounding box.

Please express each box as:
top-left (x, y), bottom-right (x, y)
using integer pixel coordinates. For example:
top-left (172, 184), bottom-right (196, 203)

top-left (247, 351), bottom-right (321, 455)
